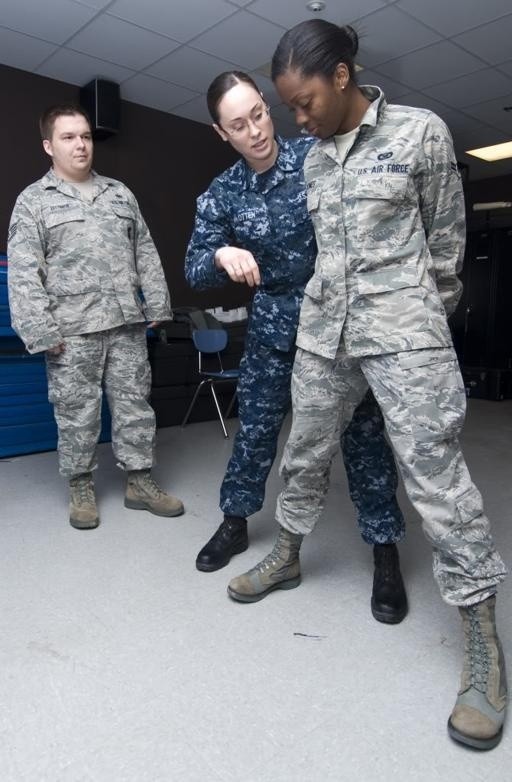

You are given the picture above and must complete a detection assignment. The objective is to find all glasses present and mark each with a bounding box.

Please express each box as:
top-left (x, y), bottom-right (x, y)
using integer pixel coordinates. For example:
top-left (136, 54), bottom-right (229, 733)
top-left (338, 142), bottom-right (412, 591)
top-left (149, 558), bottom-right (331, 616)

top-left (222, 105), bottom-right (270, 140)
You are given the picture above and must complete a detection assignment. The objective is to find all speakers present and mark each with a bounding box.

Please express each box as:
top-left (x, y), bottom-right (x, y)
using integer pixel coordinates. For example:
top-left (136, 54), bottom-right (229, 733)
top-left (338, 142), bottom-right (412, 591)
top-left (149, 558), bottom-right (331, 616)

top-left (80, 77), bottom-right (122, 140)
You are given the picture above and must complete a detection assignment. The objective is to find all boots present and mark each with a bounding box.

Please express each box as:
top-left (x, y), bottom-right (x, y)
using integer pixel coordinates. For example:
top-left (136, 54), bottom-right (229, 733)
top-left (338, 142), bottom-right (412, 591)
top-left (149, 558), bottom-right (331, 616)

top-left (70, 471), bottom-right (99, 531)
top-left (227, 530), bottom-right (304, 602)
top-left (448, 597), bottom-right (507, 750)
top-left (196, 517), bottom-right (249, 570)
top-left (122, 471), bottom-right (185, 518)
top-left (370, 543), bottom-right (409, 622)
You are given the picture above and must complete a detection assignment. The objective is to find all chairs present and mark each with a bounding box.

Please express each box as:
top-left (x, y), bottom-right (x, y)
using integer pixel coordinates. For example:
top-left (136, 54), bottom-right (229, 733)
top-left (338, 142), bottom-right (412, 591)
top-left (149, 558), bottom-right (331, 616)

top-left (182, 328), bottom-right (244, 439)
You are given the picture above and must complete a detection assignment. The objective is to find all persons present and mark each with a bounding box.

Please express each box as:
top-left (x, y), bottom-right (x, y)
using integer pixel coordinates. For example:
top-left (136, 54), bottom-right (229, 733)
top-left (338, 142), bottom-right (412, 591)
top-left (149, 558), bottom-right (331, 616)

top-left (8, 104), bottom-right (186, 529)
top-left (227, 18), bottom-right (509, 752)
top-left (184, 70), bottom-right (411, 625)
